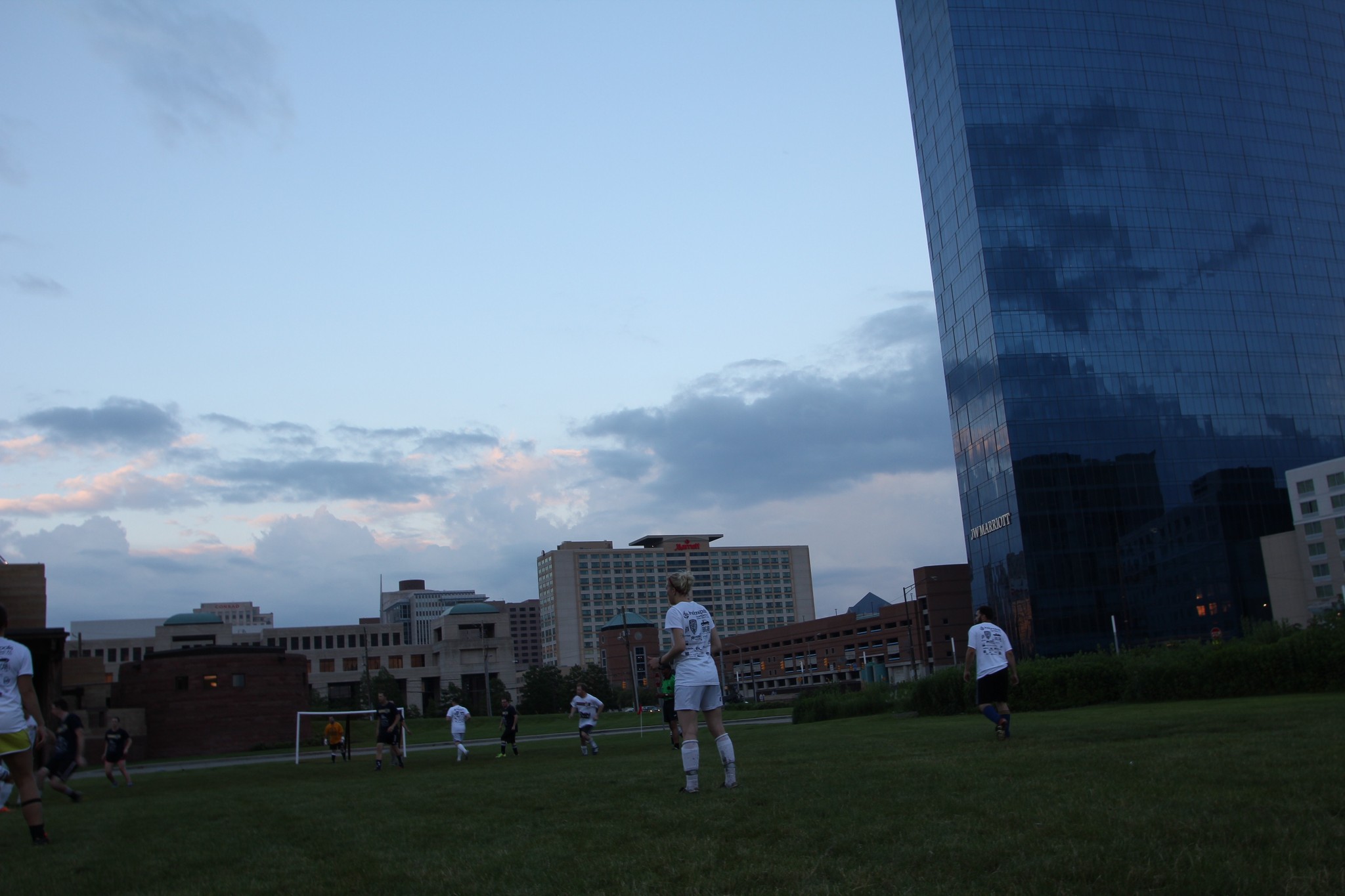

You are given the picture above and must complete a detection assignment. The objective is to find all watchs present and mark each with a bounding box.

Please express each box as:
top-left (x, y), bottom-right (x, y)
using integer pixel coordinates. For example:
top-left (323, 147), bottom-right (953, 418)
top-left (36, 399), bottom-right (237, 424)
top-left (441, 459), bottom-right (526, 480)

top-left (657, 656), bottom-right (665, 665)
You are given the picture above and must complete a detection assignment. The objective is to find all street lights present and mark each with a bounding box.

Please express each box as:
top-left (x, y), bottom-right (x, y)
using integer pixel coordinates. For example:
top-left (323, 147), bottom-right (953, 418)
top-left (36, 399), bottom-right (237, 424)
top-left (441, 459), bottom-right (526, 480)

top-left (902, 575), bottom-right (938, 681)
top-left (807, 633), bottom-right (822, 685)
top-left (724, 642), bottom-right (745, 703)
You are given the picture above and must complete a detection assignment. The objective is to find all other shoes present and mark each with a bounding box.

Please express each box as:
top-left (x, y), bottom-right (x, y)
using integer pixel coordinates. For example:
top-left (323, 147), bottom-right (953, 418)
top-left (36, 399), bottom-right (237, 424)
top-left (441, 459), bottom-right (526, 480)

top-left (112, 781), bottom-right (118, 788)
top-left (457, 760), bottom-right (462, 763)
top-left (32, 832), bottom-right (50, 846)
top-left (396, 765), bottom-right (404, 770)
top-left (71, 790), bottom-right (82, 803)
top-left (464, 750), bottom-right (470, 760)
top-left (127, 781), bottom-right (133, 787)
top-left (671, 733), bottom-right (683, 750)
top-left (331, 762), bottom-right (335, 765)
top-left (344, 759), bottom-right (348, 762)
top-left (370, 768), bottom-right (383, 772)
top-left (0, 806), bottom-right (12, 812)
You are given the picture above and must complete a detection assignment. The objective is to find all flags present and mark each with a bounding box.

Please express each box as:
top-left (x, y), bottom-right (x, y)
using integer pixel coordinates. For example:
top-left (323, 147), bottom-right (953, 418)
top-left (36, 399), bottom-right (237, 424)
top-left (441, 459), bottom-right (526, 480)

top-left (637, 703), bottom-right (643, 715)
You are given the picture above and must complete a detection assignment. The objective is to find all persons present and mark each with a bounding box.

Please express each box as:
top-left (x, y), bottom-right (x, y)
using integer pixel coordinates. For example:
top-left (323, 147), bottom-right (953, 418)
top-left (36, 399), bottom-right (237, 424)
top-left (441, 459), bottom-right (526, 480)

top-left (495, 696), bottom-right (520, 759)
top-left (654, 663), bottom-right (684, 751)
top-left (645, 570), bottom-right (741, 798)
top-left (385, 700), bottom-right (412, 765)
top-left (567, 681), bottom-right (604, 757)
top-left (759, 692), bottom-right (765, 703)
top-left (0, 607), bottom-right (59, 846)
top-left (324, 715), bottom-right (349, 764)
top-left (33, 698), bottom-right (86, 804)
top-left (446, 695), bottom-right (471, 763)
top-left (18, 700), bottom-right (39, 764)
top-left (101, 717), bottom-right (134, 790)
top-left (0, 757), bottom-right (18, 813)
top-left (372, 690), bottom-right (405, 772)
top-left (963, 605), bottom-right (1021, 741)
top-left (771, 690), bottom-right (777, 695)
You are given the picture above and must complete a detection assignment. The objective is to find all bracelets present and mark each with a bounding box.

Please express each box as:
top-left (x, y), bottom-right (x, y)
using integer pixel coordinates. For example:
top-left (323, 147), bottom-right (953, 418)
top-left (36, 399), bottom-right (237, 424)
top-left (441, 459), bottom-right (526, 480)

top-left (665, 693), bottom-right (668, 697)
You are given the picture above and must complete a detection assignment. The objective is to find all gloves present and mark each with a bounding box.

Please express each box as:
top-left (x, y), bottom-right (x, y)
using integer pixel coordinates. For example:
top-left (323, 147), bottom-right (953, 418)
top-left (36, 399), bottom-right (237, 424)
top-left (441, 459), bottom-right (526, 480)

top-left (324, 738), bottom-right (327, 745)
top-left (341, 736), bottom-right (345, 743)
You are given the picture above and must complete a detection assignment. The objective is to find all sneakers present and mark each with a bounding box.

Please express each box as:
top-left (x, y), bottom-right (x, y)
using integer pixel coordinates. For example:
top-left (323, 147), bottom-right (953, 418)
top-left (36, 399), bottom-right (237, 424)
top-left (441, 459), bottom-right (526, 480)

top-left (996, 718), bottom-right (1007, 741)
top-left (679, 787), bottom-right (700, 795)
top-left (592, 746), bottom-right (599, 755)
top-left (719, 782), bottom-right (738, 790)
top-left (495, 753), bottom-right (506, 758)
